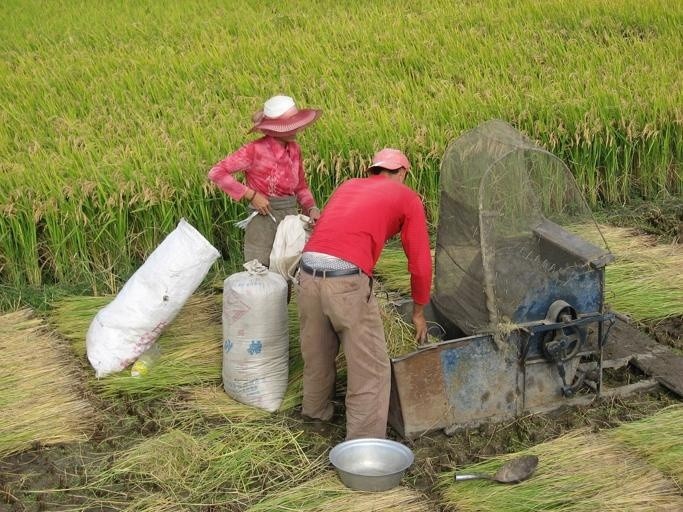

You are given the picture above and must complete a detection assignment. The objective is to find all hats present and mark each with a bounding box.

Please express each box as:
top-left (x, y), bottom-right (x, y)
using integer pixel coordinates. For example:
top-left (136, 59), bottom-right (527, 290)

top-left (247, 94), bottom-right (323, 139)
top-left (362, 148), bottom-right (412, 174)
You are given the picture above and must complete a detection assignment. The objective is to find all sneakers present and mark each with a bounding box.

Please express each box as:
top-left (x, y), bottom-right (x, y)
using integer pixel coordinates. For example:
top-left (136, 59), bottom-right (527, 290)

top-left (309, 417), bottom-right (333, 435)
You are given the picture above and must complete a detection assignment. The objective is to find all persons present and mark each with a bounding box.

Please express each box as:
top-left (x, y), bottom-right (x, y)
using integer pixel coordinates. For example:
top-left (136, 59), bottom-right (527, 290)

top-left (206, 95), bottom-right (320, 305)
top-left (295, 148), bottom-right (434, 440)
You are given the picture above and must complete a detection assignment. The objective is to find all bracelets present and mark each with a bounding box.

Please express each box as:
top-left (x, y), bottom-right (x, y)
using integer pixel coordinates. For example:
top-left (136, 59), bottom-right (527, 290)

top-left (249, 190), bottom-right (256, 202)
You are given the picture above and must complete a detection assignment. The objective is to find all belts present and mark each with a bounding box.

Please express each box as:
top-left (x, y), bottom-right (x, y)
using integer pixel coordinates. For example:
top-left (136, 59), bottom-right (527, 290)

top-left (297, 255), bottom-right (362, 279)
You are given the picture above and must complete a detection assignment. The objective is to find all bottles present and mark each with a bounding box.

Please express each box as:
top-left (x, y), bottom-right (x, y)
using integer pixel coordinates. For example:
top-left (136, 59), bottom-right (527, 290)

top-left (130, 342), bottom-right (161, 377)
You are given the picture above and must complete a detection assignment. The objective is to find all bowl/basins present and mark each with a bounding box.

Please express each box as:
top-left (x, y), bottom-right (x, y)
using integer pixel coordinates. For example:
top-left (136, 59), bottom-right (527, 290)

top-left (329, 438), bottom-right (416, 493)
